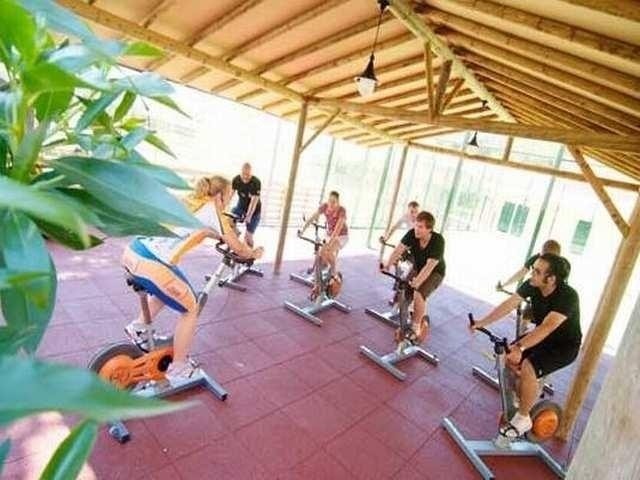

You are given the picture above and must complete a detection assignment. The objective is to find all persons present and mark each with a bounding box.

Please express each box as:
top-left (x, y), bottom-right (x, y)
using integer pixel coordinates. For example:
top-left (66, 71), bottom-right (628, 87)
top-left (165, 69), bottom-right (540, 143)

top-left (380, 211), bottom-right (445, 342)
top-left (498, 240), bottom-right (561, 334)
top-left (121, 174), bottom-right (265, 381)
top-left (381, 201), bottom-right (421, 244)
top-left (468, 253), bottom-right (582, 436)
top-left (223, 163), bottom-right (262, 269)
top-left (297, 191), bottom-right (349, 287)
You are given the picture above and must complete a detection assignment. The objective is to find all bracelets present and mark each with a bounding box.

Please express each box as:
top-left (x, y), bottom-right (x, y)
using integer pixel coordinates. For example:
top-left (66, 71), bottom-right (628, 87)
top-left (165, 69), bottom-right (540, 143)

top-left (513, 342), bottom-right (526, 353)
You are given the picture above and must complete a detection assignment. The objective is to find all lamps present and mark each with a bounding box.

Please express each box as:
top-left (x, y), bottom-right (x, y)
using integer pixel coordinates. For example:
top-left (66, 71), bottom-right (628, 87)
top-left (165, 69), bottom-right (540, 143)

top-left (466, 100), bottom-right (490, 156)
top-left (352, 0), bottom-right (391, 98)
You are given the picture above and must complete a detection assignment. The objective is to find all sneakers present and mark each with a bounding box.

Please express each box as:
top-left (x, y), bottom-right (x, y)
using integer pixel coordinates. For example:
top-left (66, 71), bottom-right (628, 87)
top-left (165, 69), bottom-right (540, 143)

top-left (125, 322), bottom-right (150, 351)
top-left (327, 269), bottom-right (335, 284)
top-left (410, 321), bottom-right (421, 339)
top-left (166, 363), bottom-right (198, 379)
top-left (499, 412), bottom-right (532, 437)
